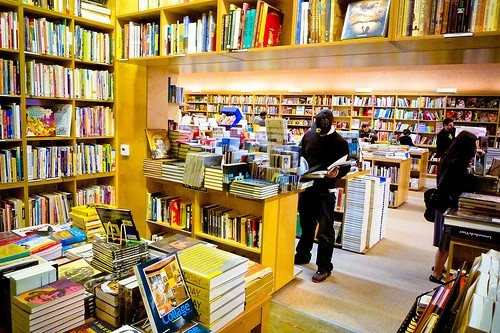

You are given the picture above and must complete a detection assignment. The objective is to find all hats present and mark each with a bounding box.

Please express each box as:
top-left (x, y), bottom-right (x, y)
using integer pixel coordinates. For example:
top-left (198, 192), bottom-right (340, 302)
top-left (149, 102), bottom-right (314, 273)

top-left (315, 112), bottom-right (332, 134)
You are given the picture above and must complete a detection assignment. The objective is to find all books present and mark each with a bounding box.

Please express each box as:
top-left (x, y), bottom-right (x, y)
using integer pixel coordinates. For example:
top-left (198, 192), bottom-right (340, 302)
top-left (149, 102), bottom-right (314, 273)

top-left (0, 0), bottom-right (500, 333)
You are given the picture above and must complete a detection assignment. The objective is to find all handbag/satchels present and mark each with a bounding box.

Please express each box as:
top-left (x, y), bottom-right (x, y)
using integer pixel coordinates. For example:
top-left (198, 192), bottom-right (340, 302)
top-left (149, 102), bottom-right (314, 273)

top-left (424, 188), bottom-right (438, 222)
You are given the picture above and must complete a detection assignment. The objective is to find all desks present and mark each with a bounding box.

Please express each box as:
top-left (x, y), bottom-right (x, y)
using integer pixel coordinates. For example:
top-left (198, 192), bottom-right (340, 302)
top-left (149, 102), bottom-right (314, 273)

top-left (216, 296), bottom-right (272, 333)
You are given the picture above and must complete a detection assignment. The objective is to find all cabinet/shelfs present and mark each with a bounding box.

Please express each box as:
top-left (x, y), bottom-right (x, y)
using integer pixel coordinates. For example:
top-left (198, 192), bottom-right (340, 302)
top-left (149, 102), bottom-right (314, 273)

top-left (350, 93), bottom-right (373, 131)
top-left (332, 95), bottom-right (353, 132)
top-left (446, 94), bottom-right (500, 150)
top-left (364, 157), bottom-right (412, 208)
top-left (408, 151), bottom-right (428, 190)
top-left (279, 188), bottom-right (303, 292)
top-left (426, 147), bottom-right (439, 178)
top-left (116, 0), bottom-right (500, 66)
top-left (372, 94), bottom-right (396, 144)
top-left (280, 94), bottom-right (313, 135)
top-left (445, 241), bottom-right (490, 286)
top-left (296, 171), bottom-right (370, 247)
top-left (145, 177), bottom-right (278, 295)
top-left (416, 95), bottom-right (446, 148)
top-left (0, 0), bottom-right (114, 227)
top-left (209, 93), bottom-right (280, 126)
top-left (183, 91), bottom-right (208, 118)
top-left (313, 94), bottom-right (332, 127)
top-left (393, 94), bottom-right (420, 146)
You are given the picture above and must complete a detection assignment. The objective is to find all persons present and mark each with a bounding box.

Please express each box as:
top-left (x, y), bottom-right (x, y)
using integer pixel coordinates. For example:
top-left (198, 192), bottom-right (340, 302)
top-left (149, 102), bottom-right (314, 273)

top-left (400, 129), bottom-right (415, 146)
top-left (253, 112), bottom-right (267, 126)
top-left (437, 118), bottom-right (456, 164)
top-left (295, 109), bottom-right (351, 283)
top-left (358, 124), bottom-right (378, 144)
top-left (428, 131), bottom-right (485, 285)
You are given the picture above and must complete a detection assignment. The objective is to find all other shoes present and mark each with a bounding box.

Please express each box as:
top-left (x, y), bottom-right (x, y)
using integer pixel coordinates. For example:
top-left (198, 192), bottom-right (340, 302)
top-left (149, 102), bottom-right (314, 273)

top-left (429, 274), bottom-right (446, 284)
top-left (295, 254), bottom-right (310, 264)
top-left (312, 270), bottom-right (331, 283)
top-left (432, 266), bottom-right (447, 273)
